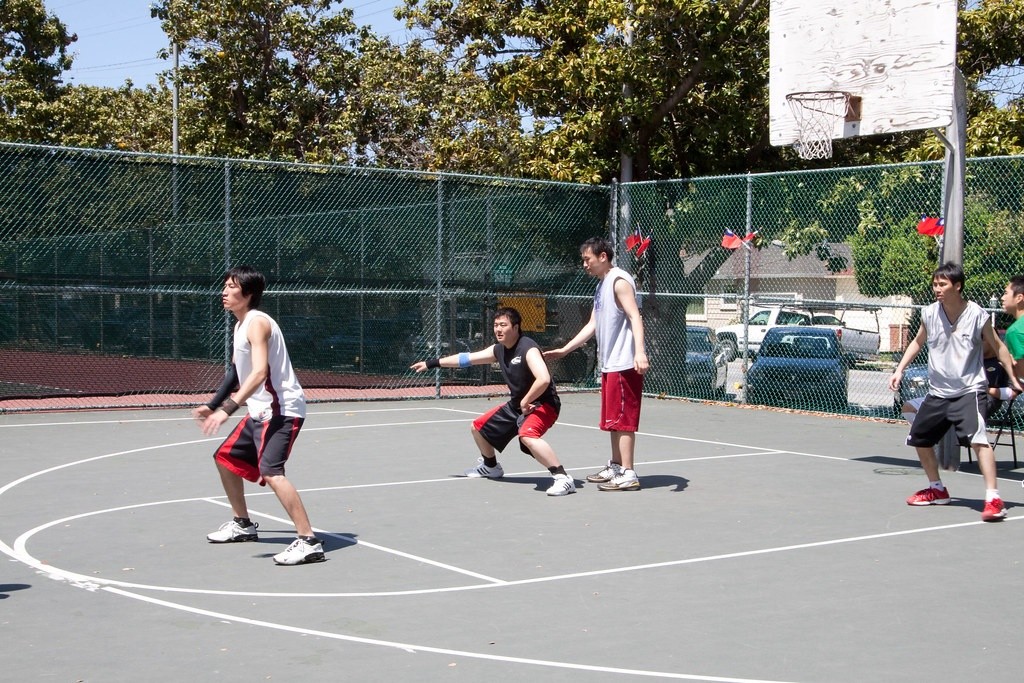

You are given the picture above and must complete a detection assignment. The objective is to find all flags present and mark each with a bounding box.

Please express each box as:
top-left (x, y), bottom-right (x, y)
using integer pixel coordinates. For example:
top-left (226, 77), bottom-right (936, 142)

top-left (721, 226), bottom-right (763, 248)
top-left (915, 215), bottom-right (945, 235)
top-left (624, 223), bottom-right (652, 257)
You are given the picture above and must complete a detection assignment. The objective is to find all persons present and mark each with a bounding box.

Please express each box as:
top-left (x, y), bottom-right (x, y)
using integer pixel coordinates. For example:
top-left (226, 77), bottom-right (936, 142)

top-left (410, 308), bottom-right (576, 495)
top-left (192, 266), bottom-right (326, 565)
top-left (542, 238), bottom-right (649, 492)
top-left (890, 261), bottom-right (1024, 520)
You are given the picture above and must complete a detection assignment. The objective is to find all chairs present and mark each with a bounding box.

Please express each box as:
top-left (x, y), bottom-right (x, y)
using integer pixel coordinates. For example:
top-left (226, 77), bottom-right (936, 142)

top-left (688, 337), bottom-right (705, 352)
top-left (963, 389), bottom-right (1022, 474)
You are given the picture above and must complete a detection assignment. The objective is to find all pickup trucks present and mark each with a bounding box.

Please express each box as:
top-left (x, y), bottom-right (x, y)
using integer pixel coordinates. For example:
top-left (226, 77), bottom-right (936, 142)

top-left (716, 305), bottom-right (883, 368)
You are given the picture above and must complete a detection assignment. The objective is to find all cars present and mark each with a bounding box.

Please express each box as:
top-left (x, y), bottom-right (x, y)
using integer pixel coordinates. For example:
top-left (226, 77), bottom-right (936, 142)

top-left (894, 334), bottom-right (1024, 425)
top-left (683, 326), bottom-right (727, 399)
top-left (1, 296), bottom-right (480, 375)
top-left (488, 336), bottom-right (598, 385)
top-left (746, 325), bottom-right (856, 413)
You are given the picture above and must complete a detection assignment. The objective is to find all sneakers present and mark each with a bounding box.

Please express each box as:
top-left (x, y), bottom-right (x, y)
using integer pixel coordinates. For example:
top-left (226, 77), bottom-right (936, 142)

top-left (597, 468), bottom-right (641, 491)
top-left (464, 458), bottom-right (504, 479)
top-left (981, 498), bottom-right (1008, 522)
top-left (546, 472), bottom-right (577, 496)
top-left (906, 487), bottom-right (951, 506)
top-left (273, 539), bottom-right (327, 565)
top-left (586, 463), bottom-right (622, 483)
top-left (207, 520), bottom-right (259, 543)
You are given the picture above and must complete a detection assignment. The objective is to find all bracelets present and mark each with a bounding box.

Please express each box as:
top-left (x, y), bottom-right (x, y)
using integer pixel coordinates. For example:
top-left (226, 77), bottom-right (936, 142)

top-left (426, 359), bottom-right (441, 370)
top-left (221, 398), bottom-right (239, 416)
